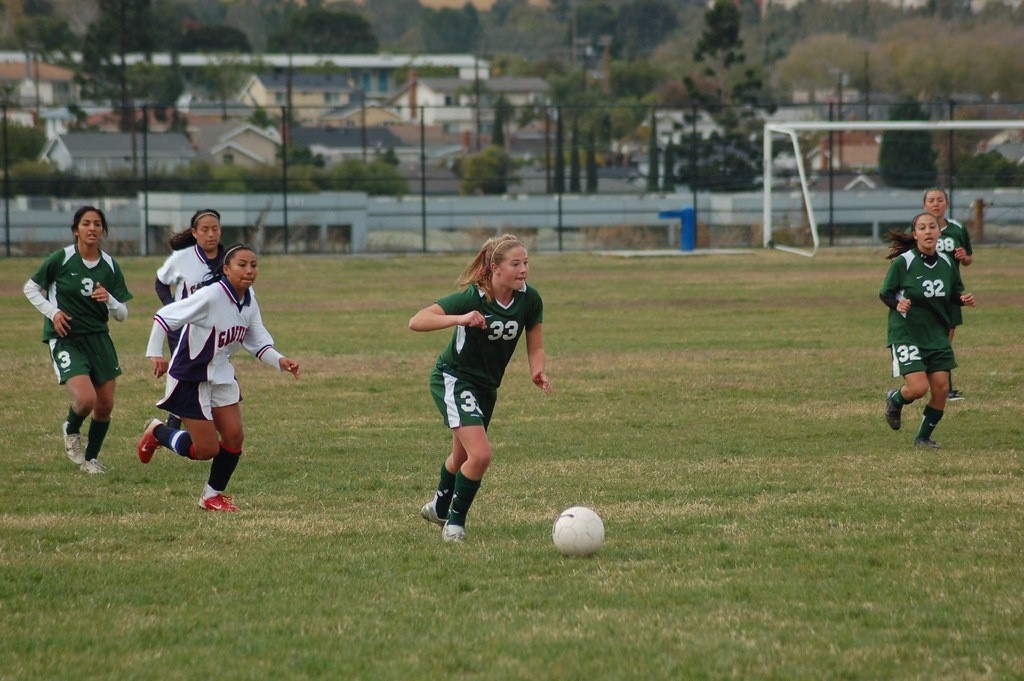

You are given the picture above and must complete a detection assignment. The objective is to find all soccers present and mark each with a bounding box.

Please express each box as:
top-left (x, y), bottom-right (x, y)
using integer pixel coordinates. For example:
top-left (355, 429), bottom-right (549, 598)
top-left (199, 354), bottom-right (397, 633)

top-left (554, 505), bottom-right (606, 556)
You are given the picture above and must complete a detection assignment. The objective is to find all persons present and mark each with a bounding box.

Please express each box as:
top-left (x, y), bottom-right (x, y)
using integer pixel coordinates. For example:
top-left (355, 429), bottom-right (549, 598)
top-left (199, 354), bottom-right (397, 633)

top-left (22, 204), bottom-right (135, 476)
top-left (409, 232), bottom-right (553, 544)
top-left (878, 211), bottom-right (975, 448)
top-left (903, 186), bottom-right (975, 398)
top-left (138, 208), bottom-right (301, 513)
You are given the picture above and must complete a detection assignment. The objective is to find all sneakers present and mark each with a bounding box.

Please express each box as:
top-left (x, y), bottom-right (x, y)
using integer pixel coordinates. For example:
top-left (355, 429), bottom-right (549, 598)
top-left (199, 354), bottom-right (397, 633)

top-left (138, 418), bottom-right (165, 464)
top-left (197, 493), bottom-right (238, 513)
top-left (914, 437), bottom-right (941, 450)
top-left (885, 390), bottom-right (901, 430)
top-left (442, 519), bottom-right (465, 544)
top-left (421, 502), bottom-right (451, 527)
top-left (62, 422), bottom-right (86, 464)
top-left (81, 459), bottom-right (105, 474)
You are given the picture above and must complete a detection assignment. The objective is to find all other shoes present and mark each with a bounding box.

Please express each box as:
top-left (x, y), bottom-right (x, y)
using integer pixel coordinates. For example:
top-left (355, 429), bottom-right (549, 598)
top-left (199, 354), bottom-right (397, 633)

top-left (947, 390), bottom-right (963, 400)
top-left (166, 413), bottom-right (181, 431)
